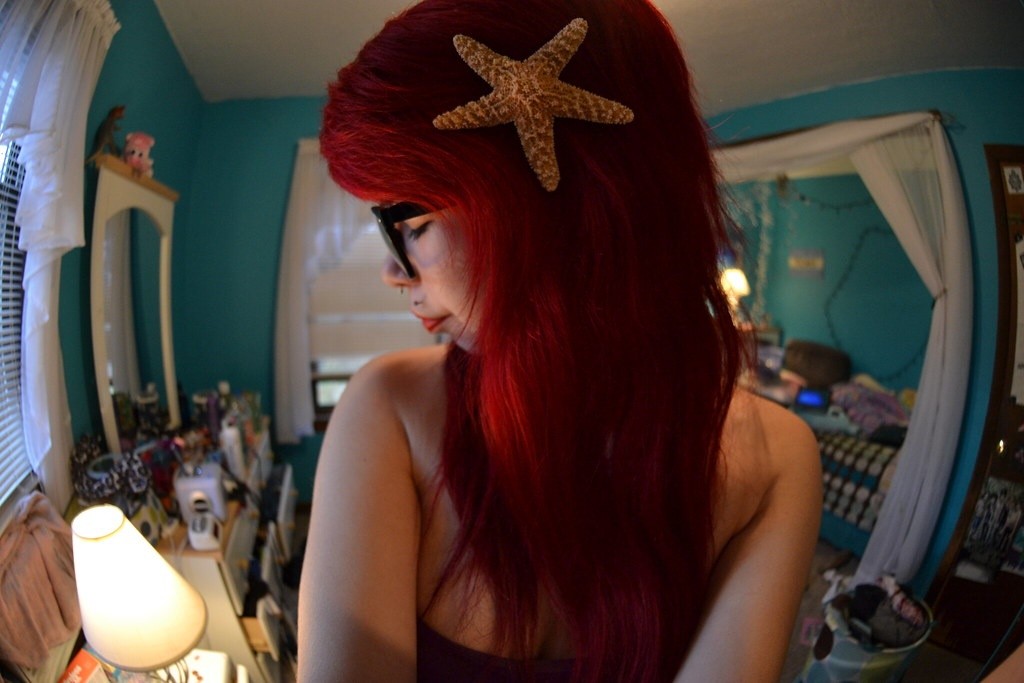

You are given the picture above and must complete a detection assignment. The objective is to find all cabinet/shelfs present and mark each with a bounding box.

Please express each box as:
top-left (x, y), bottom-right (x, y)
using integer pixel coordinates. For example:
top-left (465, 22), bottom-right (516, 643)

top-left (64, 432), bottom-right (299, 683)
top-left (156, 649), bottom-right (250, 683)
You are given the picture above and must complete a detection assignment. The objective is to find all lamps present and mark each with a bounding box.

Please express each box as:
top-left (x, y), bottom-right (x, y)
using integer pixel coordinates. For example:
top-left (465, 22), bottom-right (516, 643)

top-left (70, 503), bottom-right (209, 683)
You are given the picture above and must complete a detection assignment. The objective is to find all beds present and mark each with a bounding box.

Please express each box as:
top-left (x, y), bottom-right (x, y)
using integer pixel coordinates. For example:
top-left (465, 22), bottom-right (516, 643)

top-left (741, 324), bottom-right (1024, 582)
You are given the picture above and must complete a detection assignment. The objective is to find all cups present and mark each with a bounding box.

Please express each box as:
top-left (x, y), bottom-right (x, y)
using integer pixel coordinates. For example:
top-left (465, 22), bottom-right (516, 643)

top-left (137, 394), bottom-right (160, 414)
top-left (192, 391), bottom-right (219, 422)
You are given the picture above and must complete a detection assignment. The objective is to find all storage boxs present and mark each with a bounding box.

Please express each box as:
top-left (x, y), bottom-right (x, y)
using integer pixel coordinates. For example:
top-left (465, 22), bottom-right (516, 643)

top-left (58, 648), bottom-right (109, 683)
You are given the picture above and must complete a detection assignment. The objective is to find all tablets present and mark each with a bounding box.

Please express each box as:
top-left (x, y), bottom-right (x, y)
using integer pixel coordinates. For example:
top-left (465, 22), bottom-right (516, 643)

top-left (794, 387), bottom-right (830, 415)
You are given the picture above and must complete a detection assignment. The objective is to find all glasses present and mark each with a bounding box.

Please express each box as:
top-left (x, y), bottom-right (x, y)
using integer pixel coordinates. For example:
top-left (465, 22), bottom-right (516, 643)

top-left (370, 195), bottom-right (458, 279)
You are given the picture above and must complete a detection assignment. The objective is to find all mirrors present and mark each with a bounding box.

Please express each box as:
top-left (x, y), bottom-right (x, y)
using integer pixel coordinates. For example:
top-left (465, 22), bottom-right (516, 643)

top-left (89, 154), bottom-right (182, 467)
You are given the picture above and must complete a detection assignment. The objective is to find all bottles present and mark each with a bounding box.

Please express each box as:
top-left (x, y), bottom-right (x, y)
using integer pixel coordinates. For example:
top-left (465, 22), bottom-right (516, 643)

top-left (218, 418), bottom-right (245, 476)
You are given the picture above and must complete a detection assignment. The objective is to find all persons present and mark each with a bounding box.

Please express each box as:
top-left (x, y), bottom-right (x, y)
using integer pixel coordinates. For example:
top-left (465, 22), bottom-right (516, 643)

top-left (290, 1), bottom-right (824, 682)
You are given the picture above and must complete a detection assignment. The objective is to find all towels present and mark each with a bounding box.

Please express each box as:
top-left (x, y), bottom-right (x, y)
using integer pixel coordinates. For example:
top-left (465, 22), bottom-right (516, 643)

top-left (0, 488), bottom-right (84, 672)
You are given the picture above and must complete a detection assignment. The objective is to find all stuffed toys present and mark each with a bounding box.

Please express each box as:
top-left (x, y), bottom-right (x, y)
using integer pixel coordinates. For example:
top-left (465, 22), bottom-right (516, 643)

top-left (121, 130), bottom-right (156, 183)
